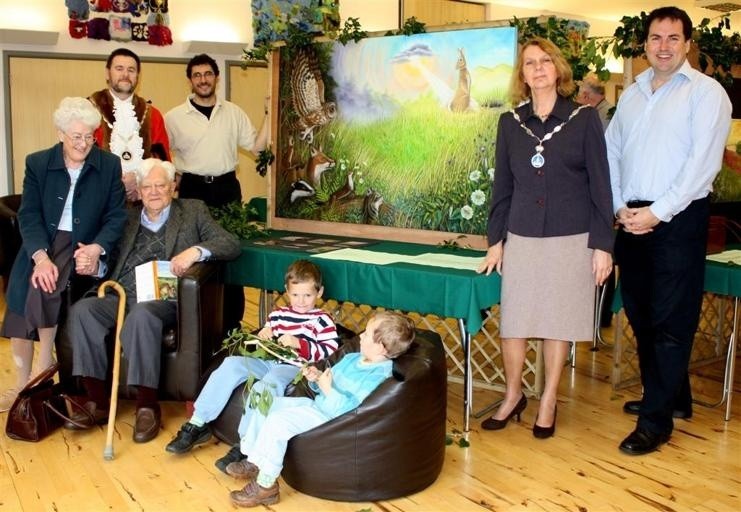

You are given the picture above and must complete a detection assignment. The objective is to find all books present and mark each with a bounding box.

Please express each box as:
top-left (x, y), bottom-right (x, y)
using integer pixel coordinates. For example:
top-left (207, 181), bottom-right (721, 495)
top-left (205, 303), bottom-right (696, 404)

top-left (134, 261), bottom-right (178, 304)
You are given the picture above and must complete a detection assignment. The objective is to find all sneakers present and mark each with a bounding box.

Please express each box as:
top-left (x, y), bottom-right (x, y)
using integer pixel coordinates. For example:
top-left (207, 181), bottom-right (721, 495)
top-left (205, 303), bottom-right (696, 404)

top-left (231, 479), bottom-right (279, 507)
top-left (226, 459), bottom-right (259, 479)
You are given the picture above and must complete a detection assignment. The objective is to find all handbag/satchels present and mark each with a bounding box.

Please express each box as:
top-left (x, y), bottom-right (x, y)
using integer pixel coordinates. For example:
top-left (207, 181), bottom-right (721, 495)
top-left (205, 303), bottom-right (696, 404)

top-left (6, 379), bottom-right (70, 442)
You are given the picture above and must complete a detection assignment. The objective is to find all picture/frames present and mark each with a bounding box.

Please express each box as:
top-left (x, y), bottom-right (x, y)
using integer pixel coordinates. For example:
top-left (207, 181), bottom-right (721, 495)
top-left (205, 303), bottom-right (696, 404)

top-left (264, 16), bottom-right (525, 253)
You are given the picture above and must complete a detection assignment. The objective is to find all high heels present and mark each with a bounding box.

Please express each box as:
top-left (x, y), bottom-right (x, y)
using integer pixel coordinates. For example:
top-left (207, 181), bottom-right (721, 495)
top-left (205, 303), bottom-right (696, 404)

top-left (482, 392), bottom-right (527, 430)
top-left (533, 404), bottom-right (557, 438)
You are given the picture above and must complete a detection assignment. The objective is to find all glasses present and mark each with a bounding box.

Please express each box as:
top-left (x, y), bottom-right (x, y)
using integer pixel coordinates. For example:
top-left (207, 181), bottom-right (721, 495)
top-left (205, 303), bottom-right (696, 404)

top-left (62, 131), bottom-right (97, 145)
top-left (191, 72), bottom-right (215, 79)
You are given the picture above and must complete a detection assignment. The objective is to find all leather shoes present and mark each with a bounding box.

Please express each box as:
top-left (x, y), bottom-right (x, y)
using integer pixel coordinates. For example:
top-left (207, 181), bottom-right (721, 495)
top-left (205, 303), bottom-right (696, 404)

top-left (167, 422), bottom-right (212, 453)
top-left (65, 402), bottom-right (111, 430)
top-left (619, 428), bottom-right (663, 454)
top-left (133, 408), bottom-right (161, 442)
top-left (216, 444), bottom-right (248, 474)
top-left (623, 400), bottom-right (691, 418)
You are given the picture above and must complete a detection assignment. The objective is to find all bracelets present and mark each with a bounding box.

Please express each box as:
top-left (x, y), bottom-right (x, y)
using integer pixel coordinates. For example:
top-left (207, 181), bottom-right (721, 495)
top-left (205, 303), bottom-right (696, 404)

top-left (36, 256), bottom-right (48, 266)
top-left (264, 110), bottom-right (269, 115)
top-left (190, 246), bottom-right (202, 262)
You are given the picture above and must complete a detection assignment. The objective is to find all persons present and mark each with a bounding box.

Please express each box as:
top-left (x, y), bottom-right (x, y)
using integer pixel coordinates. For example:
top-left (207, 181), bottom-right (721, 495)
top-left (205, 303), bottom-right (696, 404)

top-left (64, 157), bottom-right (242, 443)
top-left (603, 6), bottom-right (734, 455)
top-left (476, 38), bottom-right (613, 439)
top-left (576, 72), bottom-right (616, 131)
top-left (164, 260), bottom-right (339, 473)
top-left (89, 48), bottom-right (170, 162)
top-left (0, 96), bottom-right (126, 413)
top-left (163, 54), bottom-right (271, 331)
top-left (224, 309), bottom-right (417, 507)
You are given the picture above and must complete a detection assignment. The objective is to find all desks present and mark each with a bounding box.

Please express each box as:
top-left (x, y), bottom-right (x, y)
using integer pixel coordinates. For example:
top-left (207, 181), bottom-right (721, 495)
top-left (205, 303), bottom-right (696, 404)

top-left (609, 247), bottom-right (741, 424)
top-left (226, 228), bottom-right (499, 433)
